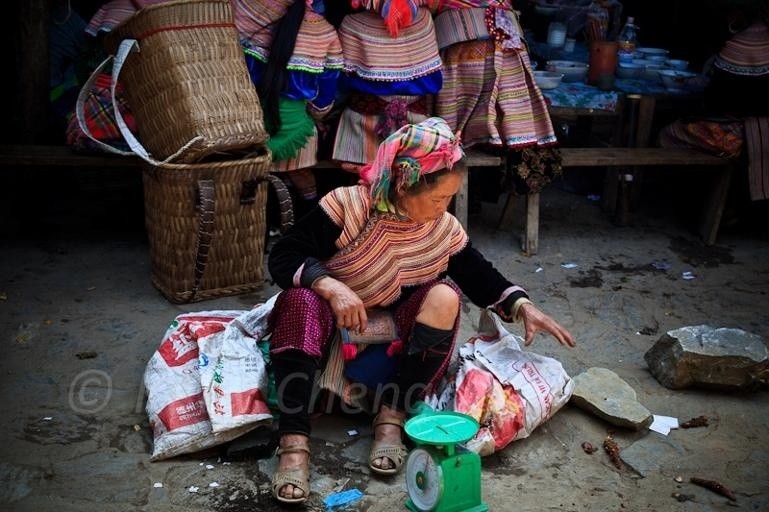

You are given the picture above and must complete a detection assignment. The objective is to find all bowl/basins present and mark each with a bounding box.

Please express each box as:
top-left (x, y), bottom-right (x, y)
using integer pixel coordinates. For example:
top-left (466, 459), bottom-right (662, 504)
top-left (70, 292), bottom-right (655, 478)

top-left (617, 47), bottom-right (698, 95)
top-left (532, 61), bottom-right (589, 89)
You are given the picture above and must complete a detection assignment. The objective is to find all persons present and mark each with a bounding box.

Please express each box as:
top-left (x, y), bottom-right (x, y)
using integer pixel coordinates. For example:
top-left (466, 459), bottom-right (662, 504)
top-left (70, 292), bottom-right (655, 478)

top-left (265, 116), bottom-right (578, 508)
top-left (649, 0), bottom-right (768, 206)
top-left (49, 1), bottom-right (562, 258)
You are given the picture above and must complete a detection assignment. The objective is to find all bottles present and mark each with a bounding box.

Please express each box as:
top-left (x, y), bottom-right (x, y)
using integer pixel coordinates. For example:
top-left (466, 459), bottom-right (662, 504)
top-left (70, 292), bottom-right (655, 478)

top-left (616, 17), bottom-right (640, 55)
top-left (565, 38), bottom-right (576, 53)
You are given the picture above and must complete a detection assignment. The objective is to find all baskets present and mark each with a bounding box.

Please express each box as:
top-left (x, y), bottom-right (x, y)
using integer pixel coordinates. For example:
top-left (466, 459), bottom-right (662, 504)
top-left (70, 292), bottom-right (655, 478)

top-left (108, 1), bottom-right (269, 163)
top-left (141, 148), bottom-right (272, 303)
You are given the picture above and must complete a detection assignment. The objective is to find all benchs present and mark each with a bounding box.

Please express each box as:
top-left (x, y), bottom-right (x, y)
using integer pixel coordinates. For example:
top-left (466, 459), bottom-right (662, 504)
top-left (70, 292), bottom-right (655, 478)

top-left (303, 144), bottom-right (738, 257)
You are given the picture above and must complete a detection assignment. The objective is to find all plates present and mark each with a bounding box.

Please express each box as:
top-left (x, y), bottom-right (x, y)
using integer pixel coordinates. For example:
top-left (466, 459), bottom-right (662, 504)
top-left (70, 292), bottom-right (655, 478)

top-left (549, 30), bottom-right (567, 48)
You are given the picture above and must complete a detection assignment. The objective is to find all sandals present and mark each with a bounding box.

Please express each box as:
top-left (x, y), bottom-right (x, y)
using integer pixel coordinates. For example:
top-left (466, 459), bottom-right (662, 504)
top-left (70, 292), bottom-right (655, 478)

top-left (271, 442), bottom-right (312, 504)
top-left (368, 413), bottom-right (406, 475)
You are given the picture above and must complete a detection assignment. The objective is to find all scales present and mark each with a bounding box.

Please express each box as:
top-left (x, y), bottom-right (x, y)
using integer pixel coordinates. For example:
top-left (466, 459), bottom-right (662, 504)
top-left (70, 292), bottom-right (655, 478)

top-left (404, 401), bottom-right (489, 512)
